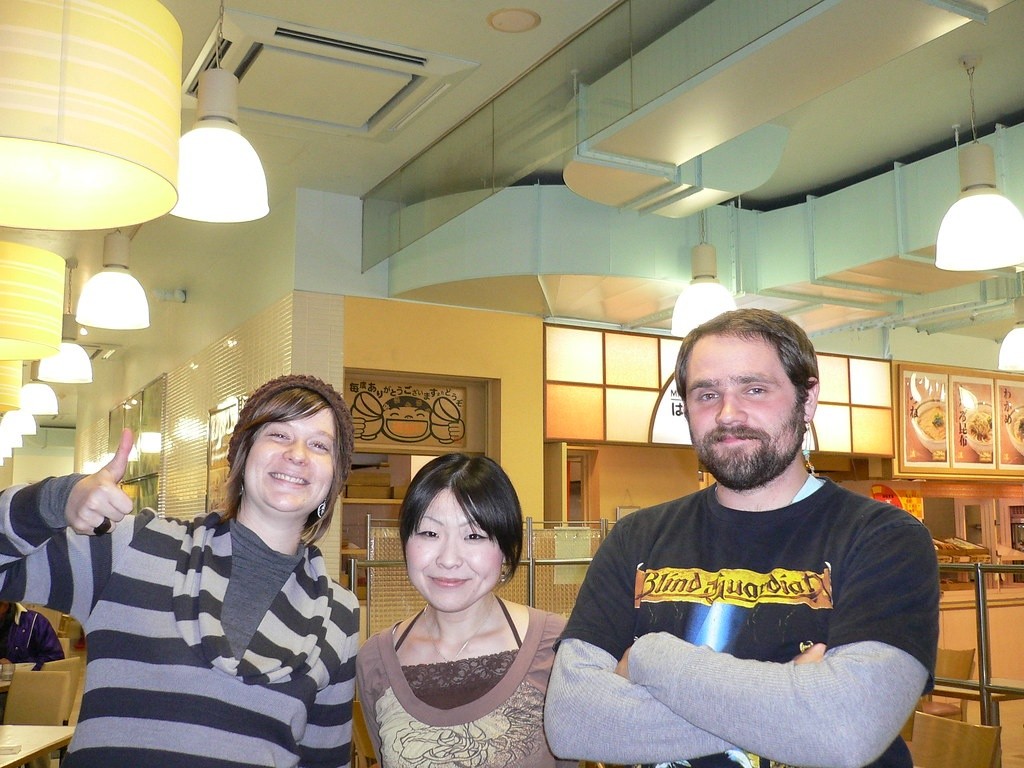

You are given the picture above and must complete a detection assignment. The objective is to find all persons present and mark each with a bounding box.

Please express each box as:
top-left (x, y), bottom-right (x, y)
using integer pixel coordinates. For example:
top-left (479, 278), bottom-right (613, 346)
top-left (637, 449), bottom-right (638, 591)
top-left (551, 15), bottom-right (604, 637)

top-left (544, 309), bottom-right (941, 768)
top-left (0, 600), bottom-right (65, 725)
top-left (356, 453), bottom-right (581, 768)
top-left (0, 376), bottom-right (361, 768)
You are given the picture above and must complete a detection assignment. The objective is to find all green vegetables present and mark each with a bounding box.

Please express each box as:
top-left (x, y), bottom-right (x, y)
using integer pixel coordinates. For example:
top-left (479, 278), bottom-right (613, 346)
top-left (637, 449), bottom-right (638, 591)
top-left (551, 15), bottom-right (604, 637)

top-left (932, 413), bottom-right (943, 427)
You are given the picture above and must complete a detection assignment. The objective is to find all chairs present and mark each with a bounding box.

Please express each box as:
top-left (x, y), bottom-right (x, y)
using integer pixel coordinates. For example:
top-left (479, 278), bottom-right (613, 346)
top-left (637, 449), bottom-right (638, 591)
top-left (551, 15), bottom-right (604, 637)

top-left (3, 604), bottom-right (86, 768)
top-left (900, 647), bottom-right (1002, 768)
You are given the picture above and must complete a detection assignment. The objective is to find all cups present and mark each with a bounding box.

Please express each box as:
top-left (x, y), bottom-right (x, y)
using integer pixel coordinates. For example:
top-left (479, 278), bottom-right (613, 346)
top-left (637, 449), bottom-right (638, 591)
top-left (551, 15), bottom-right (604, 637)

top-left (2, 664), bottom-right (15, 680)
top-left (59, 638), bottom-right (70, 658)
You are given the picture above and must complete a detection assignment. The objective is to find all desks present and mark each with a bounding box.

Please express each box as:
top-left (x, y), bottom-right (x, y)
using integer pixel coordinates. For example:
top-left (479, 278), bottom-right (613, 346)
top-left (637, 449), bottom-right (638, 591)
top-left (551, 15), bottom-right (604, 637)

top-left (929, 678), bottom-right (1024, 727)
top-left (0, 725), bottom-right (75, 768)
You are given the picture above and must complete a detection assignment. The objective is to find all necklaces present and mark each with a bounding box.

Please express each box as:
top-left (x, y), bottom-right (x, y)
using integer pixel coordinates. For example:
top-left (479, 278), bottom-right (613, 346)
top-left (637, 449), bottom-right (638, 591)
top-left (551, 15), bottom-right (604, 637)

top-left (425, 591), bottom-right (495, 663)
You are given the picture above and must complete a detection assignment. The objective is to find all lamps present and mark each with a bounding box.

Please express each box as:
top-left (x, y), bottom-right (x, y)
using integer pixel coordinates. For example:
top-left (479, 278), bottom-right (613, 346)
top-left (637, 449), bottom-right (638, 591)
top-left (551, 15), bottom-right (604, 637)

top-left (36, 257), bottom-right (93, 385)
top-left (168, 1), bottom-right (270, 224)
top-left (74, 227), bottom-right (152, 332)
top-left (1, 239), bottom-right (70, 415)
top-left (0, 361), bottom-right (60, 470)
top-left (670, 211), bottom-right (736, 337)
top-left (935, 55), bottom-right (1024, 270)
top-left (996, 272), bottom-right (1024, 372)
top-left (1, 0), bottom-right (183, 231)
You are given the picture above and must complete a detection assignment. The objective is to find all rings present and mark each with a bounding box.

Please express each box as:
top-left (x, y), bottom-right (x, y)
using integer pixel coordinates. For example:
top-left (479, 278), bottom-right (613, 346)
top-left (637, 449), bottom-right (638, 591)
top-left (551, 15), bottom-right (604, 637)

top-left (94, 516), bottom-right (111, 536)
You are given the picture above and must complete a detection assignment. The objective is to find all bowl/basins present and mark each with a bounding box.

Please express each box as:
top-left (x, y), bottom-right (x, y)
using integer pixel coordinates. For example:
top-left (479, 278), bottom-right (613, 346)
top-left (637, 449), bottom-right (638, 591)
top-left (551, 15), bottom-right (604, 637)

top-left (961, 402), bottom-right (993, 453)
top-left (0, 663), bottom-right (36, 679)
top-left (1005, 406), bottom-right (1024, 456)
top-left (911, 400), bottom-right (947, 451)
